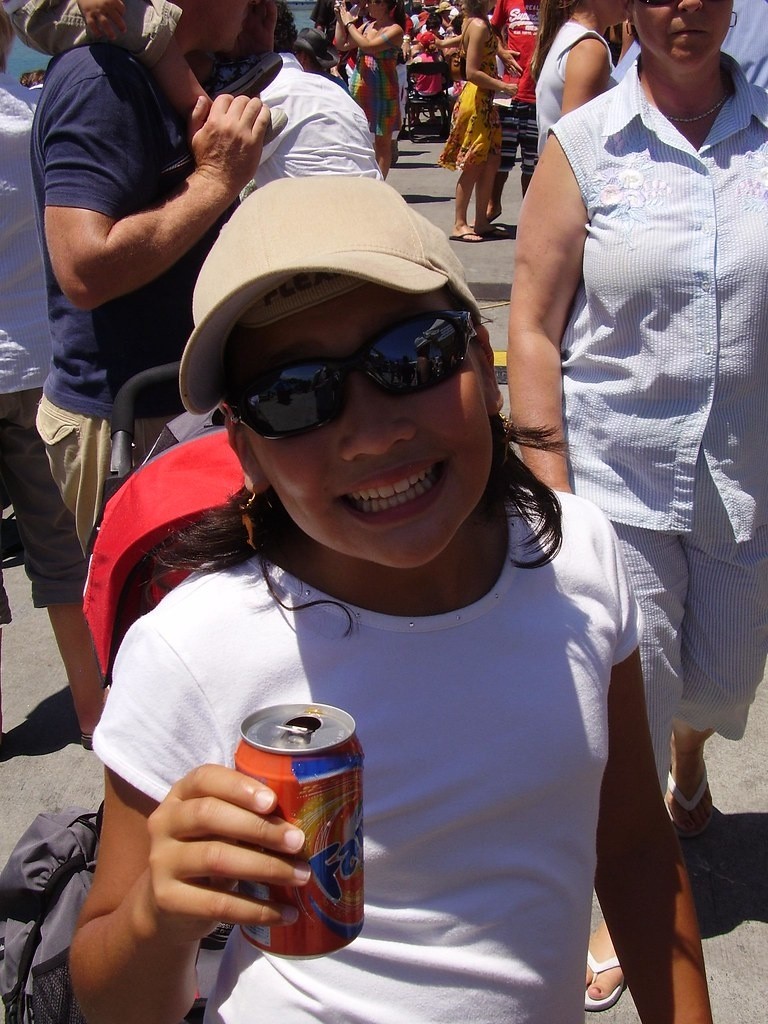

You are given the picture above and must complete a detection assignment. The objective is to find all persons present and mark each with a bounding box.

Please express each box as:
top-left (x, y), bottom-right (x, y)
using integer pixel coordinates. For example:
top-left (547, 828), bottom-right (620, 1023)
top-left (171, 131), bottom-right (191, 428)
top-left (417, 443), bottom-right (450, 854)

top-left (369, 336), bottom-right (460, 388)
top-left (0, 1), bottom-right (634, 751)
top-left (507, 1), bottom-right (768, 1012)
top-left (68, 175), bottom-right (715, 1024)
top-left (246, 365), bottom-right (339, 431)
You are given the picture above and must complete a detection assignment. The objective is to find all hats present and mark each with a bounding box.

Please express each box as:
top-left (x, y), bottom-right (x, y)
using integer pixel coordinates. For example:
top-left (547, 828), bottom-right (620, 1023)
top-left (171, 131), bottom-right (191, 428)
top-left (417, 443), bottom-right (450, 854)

top-left (417, 11), bottom-right (431, 28)
top-left (290, 26), bottom-right (339, 69)
top-left (416, 31), bottom-right (436, 47)
top-left (435, 1), bottom-right (453, 14)
top-left (178, 176), bottom-right (484, 415)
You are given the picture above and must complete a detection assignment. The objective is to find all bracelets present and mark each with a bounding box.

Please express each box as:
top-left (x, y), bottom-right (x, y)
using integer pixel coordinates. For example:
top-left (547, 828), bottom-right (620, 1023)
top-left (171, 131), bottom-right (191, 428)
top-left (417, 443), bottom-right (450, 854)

top-left (345, 22), bottom-right (354, 30)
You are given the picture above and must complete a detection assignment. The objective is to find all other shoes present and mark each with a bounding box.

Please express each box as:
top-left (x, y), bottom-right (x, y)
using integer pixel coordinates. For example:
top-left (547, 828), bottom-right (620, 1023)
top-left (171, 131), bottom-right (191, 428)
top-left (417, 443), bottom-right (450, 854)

top-left (427, 117), bottom-right (439, 125)
top-left (410, 117), bottom-right (421, 126)
top-left (81, 731), bottom-right (95, 749)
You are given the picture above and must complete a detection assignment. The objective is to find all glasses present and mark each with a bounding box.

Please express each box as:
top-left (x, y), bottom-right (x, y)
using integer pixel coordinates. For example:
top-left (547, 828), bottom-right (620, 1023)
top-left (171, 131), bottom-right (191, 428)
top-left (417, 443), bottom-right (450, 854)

top-left (222, 310), bottom-right (482, 439)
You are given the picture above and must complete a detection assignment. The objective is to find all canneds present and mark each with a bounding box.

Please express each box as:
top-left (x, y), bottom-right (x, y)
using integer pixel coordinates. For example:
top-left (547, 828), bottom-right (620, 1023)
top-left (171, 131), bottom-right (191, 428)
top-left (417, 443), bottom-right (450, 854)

top-left (232, 702), bottom-right (364, 960)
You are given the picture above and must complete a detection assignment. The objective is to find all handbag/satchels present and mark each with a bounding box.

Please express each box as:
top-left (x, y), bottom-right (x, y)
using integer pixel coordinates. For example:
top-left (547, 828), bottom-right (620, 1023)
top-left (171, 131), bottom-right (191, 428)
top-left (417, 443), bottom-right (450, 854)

top-left (450, 17), bottom-right (478, 80)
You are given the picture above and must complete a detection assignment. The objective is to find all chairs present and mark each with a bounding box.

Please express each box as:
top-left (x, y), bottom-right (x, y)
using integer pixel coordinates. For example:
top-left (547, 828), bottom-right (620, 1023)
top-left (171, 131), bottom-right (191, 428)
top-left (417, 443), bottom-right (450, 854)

top-left (402, 62), bottom-right (452, 142)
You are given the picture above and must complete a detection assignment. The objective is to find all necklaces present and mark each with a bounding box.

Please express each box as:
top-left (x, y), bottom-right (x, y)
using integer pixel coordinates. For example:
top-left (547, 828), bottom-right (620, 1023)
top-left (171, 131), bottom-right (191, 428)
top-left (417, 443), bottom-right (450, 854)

top-left (662, 94), bottom-right (727, 123)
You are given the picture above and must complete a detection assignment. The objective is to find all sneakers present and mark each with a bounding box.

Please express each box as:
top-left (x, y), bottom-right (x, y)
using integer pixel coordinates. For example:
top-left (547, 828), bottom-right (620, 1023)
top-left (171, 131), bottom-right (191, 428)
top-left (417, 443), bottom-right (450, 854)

top-left (201, 51), bottom-right (283, 102)
top-left (261, 108), bottom-right (287, 150)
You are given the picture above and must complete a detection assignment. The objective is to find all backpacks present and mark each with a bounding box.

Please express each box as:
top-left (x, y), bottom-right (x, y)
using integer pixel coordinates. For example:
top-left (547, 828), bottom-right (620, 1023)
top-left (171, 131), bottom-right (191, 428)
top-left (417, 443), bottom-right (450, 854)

top-left (0, 801), bottom-right (106, 1023)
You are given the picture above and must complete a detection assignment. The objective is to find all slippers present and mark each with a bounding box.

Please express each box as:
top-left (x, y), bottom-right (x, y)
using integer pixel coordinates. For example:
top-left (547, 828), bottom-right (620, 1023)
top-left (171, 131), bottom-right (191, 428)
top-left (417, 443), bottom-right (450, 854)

top-left (449, 233), bottom-right (484, 242)
top-left (583, 939), bottom-right (626, 1013)
top-left (469, 209), bottom-right (503, 228)
top-left (476, 228), bottom-right (510, 239)
top-left (665, 757), bottom-right (713, 838)
